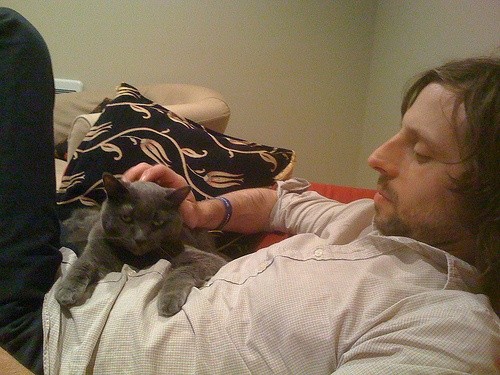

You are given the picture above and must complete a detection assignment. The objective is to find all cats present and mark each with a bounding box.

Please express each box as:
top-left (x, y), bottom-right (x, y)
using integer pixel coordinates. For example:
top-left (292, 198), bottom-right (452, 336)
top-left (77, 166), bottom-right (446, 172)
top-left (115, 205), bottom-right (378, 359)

top-left (54, 171), bottom-right (235, 318)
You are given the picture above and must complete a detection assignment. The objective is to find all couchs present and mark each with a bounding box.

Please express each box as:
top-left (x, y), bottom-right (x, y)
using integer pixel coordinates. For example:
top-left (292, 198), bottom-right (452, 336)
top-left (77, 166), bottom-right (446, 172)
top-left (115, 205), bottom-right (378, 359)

top-left (54, 84), bottom-right (230, 163)
top-left (216, 183), bottom-right (378, 258)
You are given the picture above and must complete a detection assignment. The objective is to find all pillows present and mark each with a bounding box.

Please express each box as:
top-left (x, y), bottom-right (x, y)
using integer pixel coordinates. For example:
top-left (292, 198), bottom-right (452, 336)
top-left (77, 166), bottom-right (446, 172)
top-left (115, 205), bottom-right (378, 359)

top-left (57, 81), bottom-right (295, 252)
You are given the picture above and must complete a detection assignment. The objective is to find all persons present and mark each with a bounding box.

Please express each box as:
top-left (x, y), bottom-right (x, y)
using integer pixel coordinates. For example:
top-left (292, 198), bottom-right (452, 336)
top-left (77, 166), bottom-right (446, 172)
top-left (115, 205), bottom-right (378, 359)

top-left (0, 7), bottom-right (500, 375)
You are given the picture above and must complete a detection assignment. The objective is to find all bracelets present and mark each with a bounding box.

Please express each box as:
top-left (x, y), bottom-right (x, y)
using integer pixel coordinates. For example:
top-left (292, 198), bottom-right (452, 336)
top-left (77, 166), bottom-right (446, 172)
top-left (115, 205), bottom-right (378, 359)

top-left (213, 196), bottom-right (232, 229)
